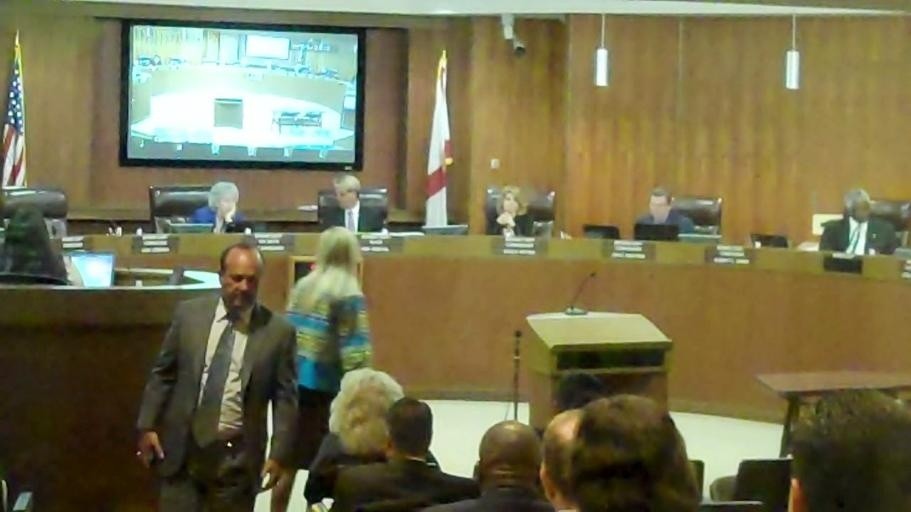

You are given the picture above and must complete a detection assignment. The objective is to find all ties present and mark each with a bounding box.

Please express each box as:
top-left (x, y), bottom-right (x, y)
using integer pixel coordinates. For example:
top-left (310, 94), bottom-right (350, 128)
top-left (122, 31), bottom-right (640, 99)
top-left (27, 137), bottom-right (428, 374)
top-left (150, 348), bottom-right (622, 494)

top-left (192, 310), bottom-right (240, 448)
top-left (348, 212), bottom-right (355, 231)
top-left (845, 222), bottom-right (860, 254)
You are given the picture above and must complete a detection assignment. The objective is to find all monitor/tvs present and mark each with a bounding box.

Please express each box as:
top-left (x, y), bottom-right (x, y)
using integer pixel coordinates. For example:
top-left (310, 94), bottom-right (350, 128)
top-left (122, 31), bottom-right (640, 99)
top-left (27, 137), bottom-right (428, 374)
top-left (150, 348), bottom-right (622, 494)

top-left (892, 247), bottom-right (911, 256)
top-left (677, 233), bottom-right (723, 244)
top-left (170, 223), bottom-right (214, 233)
top-left (119, 20), bottom-right (366, 171)
top-left (422, 223), bottom-right (469, 233)
top-left (70, 252), bottom-right (116, 287)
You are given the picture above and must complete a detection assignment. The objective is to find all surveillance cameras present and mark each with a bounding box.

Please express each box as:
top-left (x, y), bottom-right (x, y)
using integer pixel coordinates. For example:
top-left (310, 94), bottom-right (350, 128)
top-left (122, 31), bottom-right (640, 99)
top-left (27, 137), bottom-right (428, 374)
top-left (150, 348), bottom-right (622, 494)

top-left (512, 35), bottom-right (526, 56)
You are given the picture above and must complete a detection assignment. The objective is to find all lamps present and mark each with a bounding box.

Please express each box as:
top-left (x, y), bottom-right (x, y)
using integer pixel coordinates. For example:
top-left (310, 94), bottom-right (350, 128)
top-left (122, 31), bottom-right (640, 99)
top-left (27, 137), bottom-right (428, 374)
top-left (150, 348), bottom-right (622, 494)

top-left (786, 15), bottom-right (800, 92)
top-left (594, 14), bottom-right (609, 87)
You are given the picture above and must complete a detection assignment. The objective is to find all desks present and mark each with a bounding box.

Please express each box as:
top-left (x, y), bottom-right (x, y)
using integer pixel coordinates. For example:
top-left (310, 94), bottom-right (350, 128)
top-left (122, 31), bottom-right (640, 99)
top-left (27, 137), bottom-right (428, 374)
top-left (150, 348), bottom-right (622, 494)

top-left (757, 370), bottom-right (911, 458)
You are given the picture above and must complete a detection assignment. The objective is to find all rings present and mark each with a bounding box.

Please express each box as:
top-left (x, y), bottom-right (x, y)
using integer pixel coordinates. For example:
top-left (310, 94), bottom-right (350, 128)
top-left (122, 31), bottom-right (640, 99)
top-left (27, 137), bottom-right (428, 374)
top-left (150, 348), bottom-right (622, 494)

top-left (136, 451), bottom-right (141, 456)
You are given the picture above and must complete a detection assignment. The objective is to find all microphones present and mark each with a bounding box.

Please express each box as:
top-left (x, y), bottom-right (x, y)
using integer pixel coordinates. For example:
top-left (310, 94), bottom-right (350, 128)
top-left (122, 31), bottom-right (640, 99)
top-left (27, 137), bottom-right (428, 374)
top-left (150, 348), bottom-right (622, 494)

top-left (565, 270), bottom-right (597, 315)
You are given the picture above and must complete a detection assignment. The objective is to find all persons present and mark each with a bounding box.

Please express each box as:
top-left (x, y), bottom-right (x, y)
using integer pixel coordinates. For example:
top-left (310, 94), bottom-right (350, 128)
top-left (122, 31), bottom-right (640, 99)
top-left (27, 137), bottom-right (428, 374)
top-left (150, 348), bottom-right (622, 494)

top-left (485, 185), bottom-right (533, 236)
top-left (135, 244), bottom-right (298, 512)
top-left (819, 188), bottom-right (901, 274)
top-left (635, 187), bottom-right (697, 234)
top-left (270, 226), bottom-right (374, 512)
top-left (786, 389), bottom-right (910, 512)
top-left (329, 397), bottom-right (480, 512)
top-left (304, 370), bottom-right (436, 503)
top-left (422, 420), bottom-right (553, 512)
top-left (316, 174), bottom-right (391, 233)
top-left (186, 182), bottom-right (248, 233)
top-left (539, 372), bottom-right (700, 512)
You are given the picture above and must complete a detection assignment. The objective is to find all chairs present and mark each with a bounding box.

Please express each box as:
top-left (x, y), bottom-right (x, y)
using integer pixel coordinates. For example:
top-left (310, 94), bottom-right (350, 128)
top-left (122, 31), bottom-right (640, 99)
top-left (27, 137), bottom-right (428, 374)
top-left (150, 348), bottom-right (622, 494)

top-left (149, 186), bottom-right (214, 232)
top-left (867, 199), bottom-right (911, 248)
top-left (0, 188), bottom-right (68, 236)
top-left (486, 186), bottom-right (555, 235)
top-left (309, 459), bottom-right (790, 512)
top-left (318, 186), bottom-right (387, 232)
top-left (672, 195), bottom-right (722, 234)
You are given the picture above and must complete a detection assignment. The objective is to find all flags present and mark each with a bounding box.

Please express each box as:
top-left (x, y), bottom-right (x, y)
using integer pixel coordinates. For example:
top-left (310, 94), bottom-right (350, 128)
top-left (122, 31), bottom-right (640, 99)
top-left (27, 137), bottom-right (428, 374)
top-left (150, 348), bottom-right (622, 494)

top-left (424, 49), bottom-right (454, 225)
top-left (0, 28), bottom-right (27, 188)
